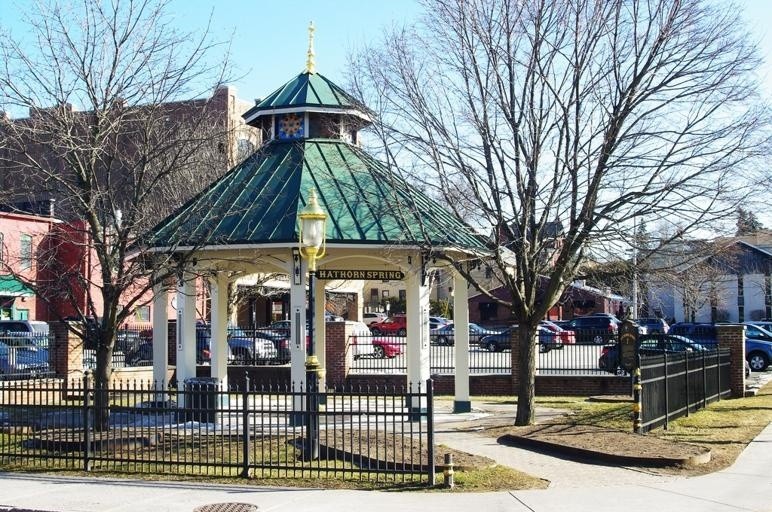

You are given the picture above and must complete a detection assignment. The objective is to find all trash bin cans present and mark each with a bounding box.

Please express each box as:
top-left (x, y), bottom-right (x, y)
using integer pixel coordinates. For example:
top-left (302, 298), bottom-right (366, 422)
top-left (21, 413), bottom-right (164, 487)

top-left (185, 378), bottom-right (218, 422)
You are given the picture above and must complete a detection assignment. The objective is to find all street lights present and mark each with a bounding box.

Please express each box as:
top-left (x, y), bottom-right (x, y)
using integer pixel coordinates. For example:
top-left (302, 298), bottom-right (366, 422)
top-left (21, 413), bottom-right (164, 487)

top-left (298, 184), bottom-right (329, 461)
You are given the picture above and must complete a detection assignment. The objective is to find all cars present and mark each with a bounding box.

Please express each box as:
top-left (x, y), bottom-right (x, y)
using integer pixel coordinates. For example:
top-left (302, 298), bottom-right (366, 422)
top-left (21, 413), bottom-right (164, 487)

top-left (598, 333), bottom-right (709, 376)
top-left (0, 336), bottom-right (49, 361)
top-left (478, 325), bottom-right (562, 353)
top-left (371, 337), bottom-right (401, 358)
top-left (0, 344), bottom-right (50, 373)
top-left (625, 319), bottom-right (646, 334)
top-left (325, 310), bottom-right (344, 321)
top-left (430, 322), bottom-right (498, 344)
top-left (127, 319), bottom-right (311, 366)
top-left (743, 321), bottom-right (772, 331)
top-left (430, 317), bottom-right (453, 330)
top-left (558, 312), bottom-right (624, 328)
top-left (537, 320), bottom-right (576, 349)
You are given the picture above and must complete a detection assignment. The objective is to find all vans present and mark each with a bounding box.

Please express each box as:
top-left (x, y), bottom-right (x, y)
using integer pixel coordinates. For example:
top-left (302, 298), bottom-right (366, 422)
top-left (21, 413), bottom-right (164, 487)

top-left (345, 320), bottom-right (373, 360)
top-left (715, 322), bottom-right (772, 341)
top-left (0, 319), bottom-right (50, 346)
top-left (560, 316), bottom-right (618, 343)
top-left (632, 317), bottom-right (668, 334)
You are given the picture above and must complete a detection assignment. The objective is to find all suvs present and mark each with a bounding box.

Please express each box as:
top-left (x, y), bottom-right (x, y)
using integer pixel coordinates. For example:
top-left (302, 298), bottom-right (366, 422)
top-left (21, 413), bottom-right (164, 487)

top-left (368, 316), bottom-right (406, 336)
top-left (363, 313), bottom-right (386, 325)
top-left (667, 322), bottom-right (772, 371)
top-left (62, 316), bottom-right (139, 351)
